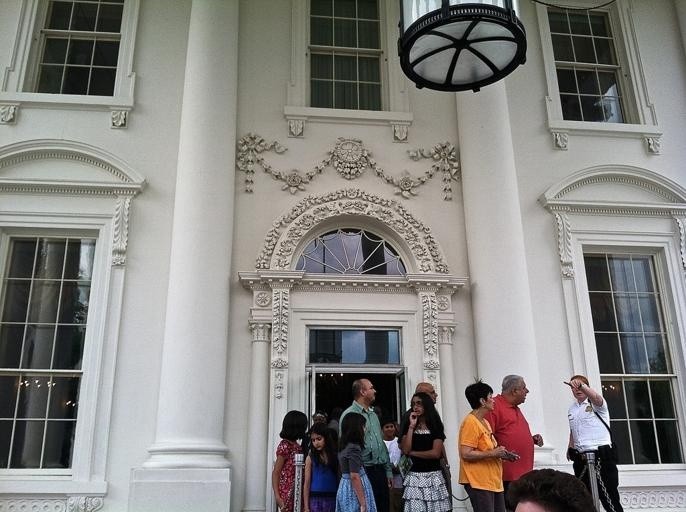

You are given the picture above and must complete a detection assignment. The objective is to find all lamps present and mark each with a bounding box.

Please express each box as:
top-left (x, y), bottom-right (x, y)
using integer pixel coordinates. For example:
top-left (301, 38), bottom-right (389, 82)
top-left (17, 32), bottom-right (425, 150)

top-left (398, 0), bottom-right (527, 93)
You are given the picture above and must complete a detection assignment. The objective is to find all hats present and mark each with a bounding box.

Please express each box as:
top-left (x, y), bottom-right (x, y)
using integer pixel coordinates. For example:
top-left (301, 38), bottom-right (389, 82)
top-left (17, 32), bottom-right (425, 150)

top-left (380, 416), bottom-right (393, 425)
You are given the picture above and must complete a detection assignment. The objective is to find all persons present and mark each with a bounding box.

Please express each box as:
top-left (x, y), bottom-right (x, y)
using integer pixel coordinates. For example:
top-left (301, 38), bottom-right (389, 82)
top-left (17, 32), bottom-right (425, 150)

top-left (508, 469), bottom-right (593, 512)
top-left (271, 378), bottom-right (453, 512)
top-left (565, 375), bottom-right (624, 512)
top-left (457, 375), bottom-right (544, 511)
